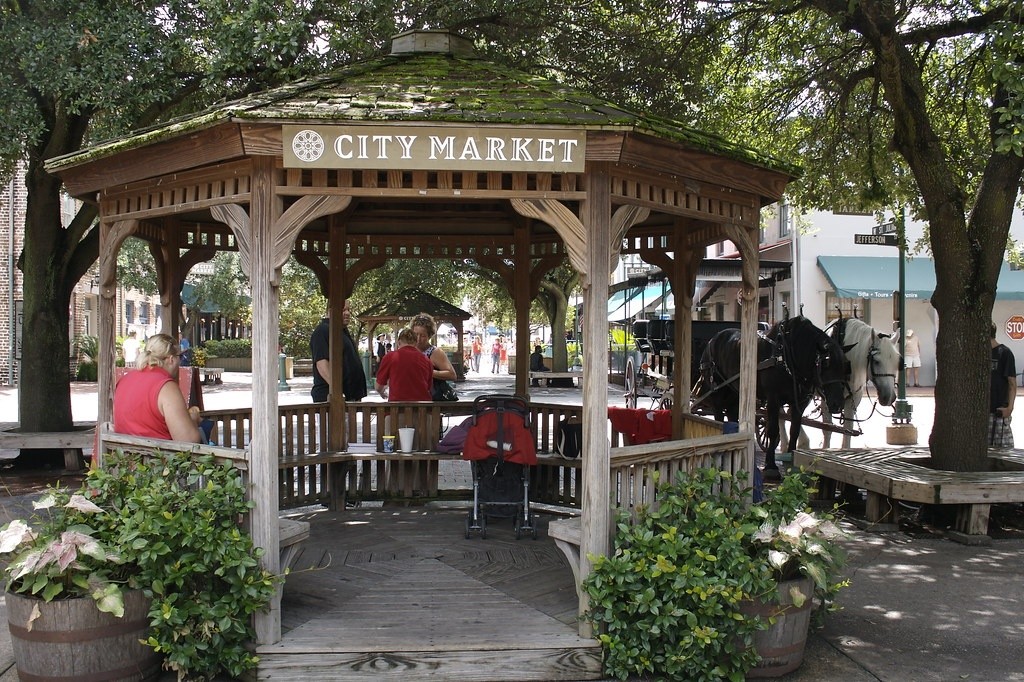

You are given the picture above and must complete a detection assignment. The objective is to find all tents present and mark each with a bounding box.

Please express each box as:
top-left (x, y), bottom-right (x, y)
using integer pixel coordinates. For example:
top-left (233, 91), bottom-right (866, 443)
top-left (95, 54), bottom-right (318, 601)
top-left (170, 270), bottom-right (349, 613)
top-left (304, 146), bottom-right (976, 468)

top-left (487, 325), bottom-right (512, 342)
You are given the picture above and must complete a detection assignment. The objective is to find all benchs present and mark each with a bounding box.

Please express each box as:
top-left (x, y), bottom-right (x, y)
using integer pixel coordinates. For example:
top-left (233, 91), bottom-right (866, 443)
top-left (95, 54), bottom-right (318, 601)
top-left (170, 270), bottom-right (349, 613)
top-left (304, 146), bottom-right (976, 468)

top-left (632, 319), bottom-right (771, 359)
top-left (529, 370), bottom-right (583, 387)
top-left (100, 400), bottom-right (747, 644)
top-left (794, 443), bottom-right (1024, 542)
top-left (1, 420), bottom-right (98, 473)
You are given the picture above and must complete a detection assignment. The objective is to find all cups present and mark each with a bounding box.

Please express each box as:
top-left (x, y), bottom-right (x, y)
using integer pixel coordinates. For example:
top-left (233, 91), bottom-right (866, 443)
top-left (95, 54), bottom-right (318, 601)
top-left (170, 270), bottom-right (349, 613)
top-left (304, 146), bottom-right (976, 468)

top-left (383, 435), bottom-right (396, 453)
top-left (399, 427), bottom-right (415, 453)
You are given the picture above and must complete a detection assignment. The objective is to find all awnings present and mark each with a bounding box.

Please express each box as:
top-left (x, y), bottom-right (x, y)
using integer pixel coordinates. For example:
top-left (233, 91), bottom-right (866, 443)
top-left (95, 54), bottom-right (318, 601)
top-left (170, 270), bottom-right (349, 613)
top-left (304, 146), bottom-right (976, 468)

top-left (608, 286), bottom-right (671, 321)
top-left (654, 280), bottom-right (724, 314)
top-left (182, 283), bottom-right (253, 313)
top-left (817, 256), bottom-right (1024, 300)
top-left (608, 287), bottom-right (639, 312)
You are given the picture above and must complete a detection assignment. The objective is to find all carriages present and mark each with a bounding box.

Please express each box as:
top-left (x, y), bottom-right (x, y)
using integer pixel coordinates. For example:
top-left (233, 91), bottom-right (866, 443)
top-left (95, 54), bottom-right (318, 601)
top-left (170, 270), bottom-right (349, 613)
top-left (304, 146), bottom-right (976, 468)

top-left (623, 256), bottom-right (903, 471)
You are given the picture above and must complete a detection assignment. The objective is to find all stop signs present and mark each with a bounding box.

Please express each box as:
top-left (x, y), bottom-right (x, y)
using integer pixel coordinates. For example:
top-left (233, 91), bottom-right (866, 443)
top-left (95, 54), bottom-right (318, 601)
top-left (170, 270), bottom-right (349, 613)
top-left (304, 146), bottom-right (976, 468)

top-left (1005, 315), bottom-right (1024, 340)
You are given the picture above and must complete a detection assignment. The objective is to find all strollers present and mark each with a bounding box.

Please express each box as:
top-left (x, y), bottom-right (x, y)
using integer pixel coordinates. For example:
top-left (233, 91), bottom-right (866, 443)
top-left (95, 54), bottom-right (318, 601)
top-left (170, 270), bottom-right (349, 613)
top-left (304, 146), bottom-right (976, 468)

top-left (463, 392), bottom-right (540, 541)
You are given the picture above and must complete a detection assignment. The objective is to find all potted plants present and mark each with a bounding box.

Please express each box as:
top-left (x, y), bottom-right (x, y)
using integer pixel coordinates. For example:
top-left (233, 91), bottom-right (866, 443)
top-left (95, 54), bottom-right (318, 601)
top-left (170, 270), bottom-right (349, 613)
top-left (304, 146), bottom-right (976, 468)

top-left (575, 456), bottom-right (853, 682)
top-left (0, 443), bottom-right (335, 682)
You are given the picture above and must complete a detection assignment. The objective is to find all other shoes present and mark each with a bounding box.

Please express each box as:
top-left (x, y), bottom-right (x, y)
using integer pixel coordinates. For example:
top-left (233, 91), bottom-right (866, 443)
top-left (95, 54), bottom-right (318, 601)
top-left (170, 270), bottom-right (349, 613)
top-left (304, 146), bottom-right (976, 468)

top-left (905, 383), bottom-right (909, 387)
top-left (913, 383), bottom-right (921, 387)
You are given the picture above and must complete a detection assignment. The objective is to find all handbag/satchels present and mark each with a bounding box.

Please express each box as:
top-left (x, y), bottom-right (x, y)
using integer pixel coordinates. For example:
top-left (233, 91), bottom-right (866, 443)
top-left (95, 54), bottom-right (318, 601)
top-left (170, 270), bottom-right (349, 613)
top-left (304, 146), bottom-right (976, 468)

top-left (433, 378), bottom-right (459, 402)
top-left (553, 422), bottom-right (583, 460)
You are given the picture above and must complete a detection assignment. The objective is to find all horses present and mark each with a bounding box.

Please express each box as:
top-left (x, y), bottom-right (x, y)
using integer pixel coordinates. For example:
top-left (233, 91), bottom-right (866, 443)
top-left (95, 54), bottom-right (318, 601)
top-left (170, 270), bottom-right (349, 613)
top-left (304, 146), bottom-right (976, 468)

top-left (702, 314), bottom-right (859, 481)
top-left (778, 315), bottom-right (901, 476)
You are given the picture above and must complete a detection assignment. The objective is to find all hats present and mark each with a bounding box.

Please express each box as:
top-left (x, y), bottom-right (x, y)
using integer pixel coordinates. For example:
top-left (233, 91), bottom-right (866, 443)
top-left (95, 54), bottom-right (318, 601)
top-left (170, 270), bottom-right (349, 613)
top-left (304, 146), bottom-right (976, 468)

top-left (905, 330), bottom-right (914, 336)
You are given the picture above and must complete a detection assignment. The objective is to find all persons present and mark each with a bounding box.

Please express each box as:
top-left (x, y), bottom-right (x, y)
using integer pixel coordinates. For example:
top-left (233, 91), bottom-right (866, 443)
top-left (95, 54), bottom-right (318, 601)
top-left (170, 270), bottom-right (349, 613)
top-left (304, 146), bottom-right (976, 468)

top-left (407, 311), bottom-right (457, 440)
top-left (375, 327), bottom-right (434, 507)
top-left (547, 333), bottom-right (552, 344)
top-left (310, 299), bottom-right (367, 508)
top-left (886, 319), bottom-right (923, 388)
top-left (989, 319), bottom-right (1017, 448)
top-left (566, 335), bottom-right (573, 344)
top-left (386, 343), bottom-right (392, 353)
top-left (490, 338), bottom-right (504, 374)
top-left (464, 336), bottom-right (485, 373)
top-left (114, 333), bottom-right (201, 444)
top-left (122, 330), bottom-right (141, 368)
top-left (530, 345), bottom-right (551, 387)
top-left (178, 333), bottom-right (190, 366)
top-left (498, 334), bottom-right (507, 365)
top-left (374, 333), bottom-right (386, 377)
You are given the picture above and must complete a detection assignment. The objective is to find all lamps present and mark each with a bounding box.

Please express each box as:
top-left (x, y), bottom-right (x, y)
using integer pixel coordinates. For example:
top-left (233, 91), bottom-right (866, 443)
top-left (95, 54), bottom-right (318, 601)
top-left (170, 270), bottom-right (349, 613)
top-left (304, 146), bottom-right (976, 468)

top-left (91, 273), bottom-right (99, 289)
